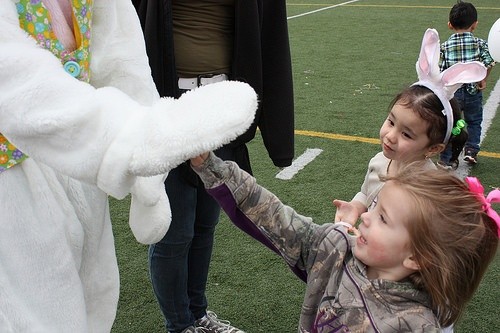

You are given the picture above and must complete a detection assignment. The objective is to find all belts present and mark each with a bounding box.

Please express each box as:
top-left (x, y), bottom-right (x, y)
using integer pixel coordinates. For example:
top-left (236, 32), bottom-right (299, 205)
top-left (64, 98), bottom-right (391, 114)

top-left (178, 74), bottom-right (227, 89)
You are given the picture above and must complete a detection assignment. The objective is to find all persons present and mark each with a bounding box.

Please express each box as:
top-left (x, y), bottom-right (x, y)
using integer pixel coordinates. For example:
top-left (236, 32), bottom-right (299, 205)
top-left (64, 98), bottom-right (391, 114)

top-left (436, 0), bottom-right (494, 169)
top-left (130, 0), bottom-right (294, 333)
top-left (188, 151), bottom-right (500, 333)
top-left (333, 28), bottom-right (487, 225)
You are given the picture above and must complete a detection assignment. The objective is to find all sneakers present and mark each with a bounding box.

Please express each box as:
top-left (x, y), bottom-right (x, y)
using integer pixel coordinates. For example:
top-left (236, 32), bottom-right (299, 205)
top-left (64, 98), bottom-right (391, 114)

top-left (463, 147), bottom-right (478, 163)
top-left (437, 160), bottom-right (452, 170)
top-left (168, 308), bottom-right (245, 333)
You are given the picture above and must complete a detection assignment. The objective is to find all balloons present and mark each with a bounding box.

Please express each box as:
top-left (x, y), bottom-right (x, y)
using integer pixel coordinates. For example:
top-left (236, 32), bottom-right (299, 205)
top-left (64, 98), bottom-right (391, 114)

top-left (488, 18), bottom-right (500, 65)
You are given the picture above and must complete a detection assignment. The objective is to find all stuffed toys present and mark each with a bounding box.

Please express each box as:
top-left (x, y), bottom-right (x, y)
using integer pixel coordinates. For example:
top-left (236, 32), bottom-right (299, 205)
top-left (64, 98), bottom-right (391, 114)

top-left (0, 0), bottom-right (259, 333)
